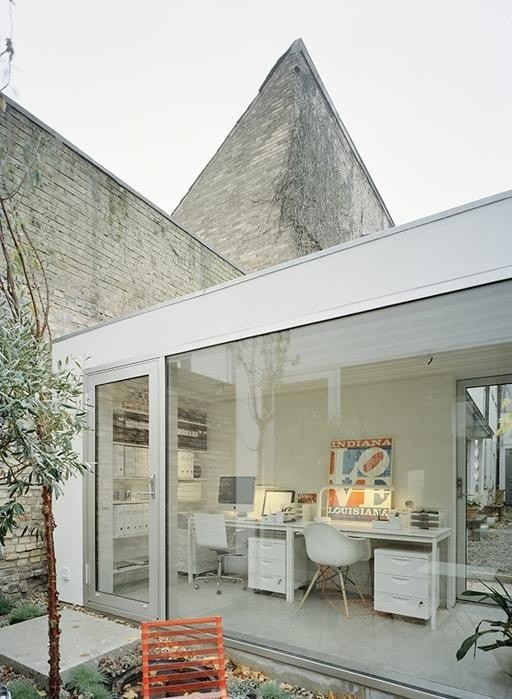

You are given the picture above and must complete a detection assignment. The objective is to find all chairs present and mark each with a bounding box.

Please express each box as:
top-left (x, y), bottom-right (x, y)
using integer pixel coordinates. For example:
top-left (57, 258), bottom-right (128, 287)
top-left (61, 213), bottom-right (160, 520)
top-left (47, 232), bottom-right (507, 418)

top-left (193, 514), bottom-right (249, 593)
top-left (300, 522), bottom-right (373, 619)
top-left (139, 616), bottom-right (228, 699)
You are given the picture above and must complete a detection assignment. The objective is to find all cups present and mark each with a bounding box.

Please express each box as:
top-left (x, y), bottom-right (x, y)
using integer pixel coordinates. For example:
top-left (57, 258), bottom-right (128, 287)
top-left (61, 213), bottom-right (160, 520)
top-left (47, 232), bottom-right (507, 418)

top-left (276, 511), bottom-right (284, 523)
top-left (390, 517), bottom-right (400, 531)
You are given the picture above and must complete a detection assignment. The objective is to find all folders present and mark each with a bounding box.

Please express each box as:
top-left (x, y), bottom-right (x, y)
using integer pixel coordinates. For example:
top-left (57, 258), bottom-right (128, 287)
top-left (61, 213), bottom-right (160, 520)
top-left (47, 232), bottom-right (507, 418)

top-left (114, 445), bottom-right (124, 478)
top-left (177, 451), bottom-right (193, 480)
top-left (114, 503), bottom-right (149, 536)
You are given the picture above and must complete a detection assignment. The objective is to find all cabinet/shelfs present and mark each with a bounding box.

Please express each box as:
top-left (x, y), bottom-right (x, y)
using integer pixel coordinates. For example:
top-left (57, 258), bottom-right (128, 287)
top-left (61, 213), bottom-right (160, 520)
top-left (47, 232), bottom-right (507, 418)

top-left (248, 536), bottom-right (307, 599)
top-left (373, 542), bottom-right (440, 625)
top-left (171, 391), bottom-right (216, 568)
top-left (113, 381), bottom-right (164, 586)
top-left (177, 507), bottom-right (217, 574)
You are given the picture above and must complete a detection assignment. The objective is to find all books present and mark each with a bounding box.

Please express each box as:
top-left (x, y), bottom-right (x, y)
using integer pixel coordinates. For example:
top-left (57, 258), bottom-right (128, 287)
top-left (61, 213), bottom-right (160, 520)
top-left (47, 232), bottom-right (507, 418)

top-left (114, 412), bottom-right (207, 477)
top-left (114, 554), bottom-right (150, 571)
top-left (113, 505), bottom-right (149, 535)
top-left (177, 482), bottom-right (202, 501)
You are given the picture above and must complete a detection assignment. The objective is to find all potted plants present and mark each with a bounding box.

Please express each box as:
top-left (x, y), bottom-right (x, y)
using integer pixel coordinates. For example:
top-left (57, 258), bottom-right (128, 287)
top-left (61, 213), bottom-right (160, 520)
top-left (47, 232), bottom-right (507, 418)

top-left (465, 496), bottom-right (481, 519)
top-left (454, 575), bottom-right (512, 678)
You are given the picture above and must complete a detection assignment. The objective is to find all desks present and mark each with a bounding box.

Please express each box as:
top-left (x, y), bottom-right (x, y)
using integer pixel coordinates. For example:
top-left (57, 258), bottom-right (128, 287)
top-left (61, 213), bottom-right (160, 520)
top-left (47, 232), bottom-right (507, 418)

top-left (289, 518), bottom-right (455, 630)
top-left (180, 510), bottom-right (312, 605)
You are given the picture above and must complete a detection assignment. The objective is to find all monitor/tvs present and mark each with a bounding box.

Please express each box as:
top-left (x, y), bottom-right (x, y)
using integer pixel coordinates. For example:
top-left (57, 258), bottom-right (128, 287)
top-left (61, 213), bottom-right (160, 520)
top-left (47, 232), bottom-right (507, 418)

top-left (217, 476), bottom-right (256, 519)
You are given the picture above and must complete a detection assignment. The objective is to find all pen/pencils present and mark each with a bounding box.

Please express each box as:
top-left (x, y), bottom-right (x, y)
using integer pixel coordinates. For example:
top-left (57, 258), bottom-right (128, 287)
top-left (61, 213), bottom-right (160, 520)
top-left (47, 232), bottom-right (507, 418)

top-left (297, 492), bottom-right (318, 505)
top-left (281, 506), bottom-right (295, 512)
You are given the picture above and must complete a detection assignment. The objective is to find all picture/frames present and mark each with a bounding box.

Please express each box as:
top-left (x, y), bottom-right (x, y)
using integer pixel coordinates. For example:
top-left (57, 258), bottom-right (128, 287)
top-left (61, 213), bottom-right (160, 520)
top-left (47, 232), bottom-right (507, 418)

top-left (261, 489), bottom-right (295, 517)
